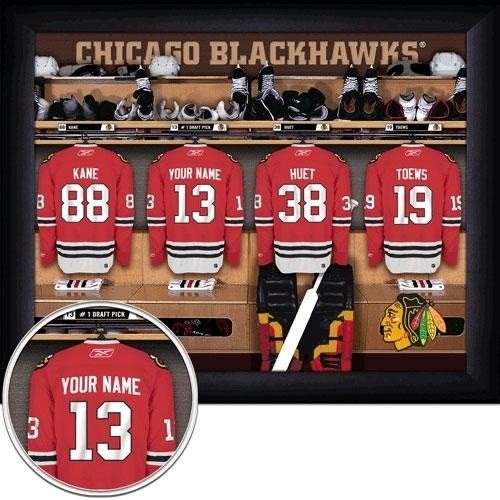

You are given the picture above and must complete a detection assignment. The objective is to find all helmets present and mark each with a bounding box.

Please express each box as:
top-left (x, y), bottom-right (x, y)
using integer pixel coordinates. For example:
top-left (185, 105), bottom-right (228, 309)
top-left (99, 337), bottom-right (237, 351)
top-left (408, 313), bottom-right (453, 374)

top-left (35, 56), bottom-right (59, 79)
top-left (430, 51), bottom-right (466, 78)
top-left (149, 55), bottom-right (180, 76)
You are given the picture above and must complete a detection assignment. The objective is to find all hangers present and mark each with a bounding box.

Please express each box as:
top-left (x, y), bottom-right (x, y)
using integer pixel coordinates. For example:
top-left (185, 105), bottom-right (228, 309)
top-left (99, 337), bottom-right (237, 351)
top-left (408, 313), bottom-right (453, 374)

top-left (402, 129), bottom-right (424, 146)
top-left (84, 318), bottom-right (120, 345)
top-left (182, 128), bottom-right (207, 146)
top-left (290, 132), bottom-right (315, 145)
top-left (70, 132), bottom-right (96, 145)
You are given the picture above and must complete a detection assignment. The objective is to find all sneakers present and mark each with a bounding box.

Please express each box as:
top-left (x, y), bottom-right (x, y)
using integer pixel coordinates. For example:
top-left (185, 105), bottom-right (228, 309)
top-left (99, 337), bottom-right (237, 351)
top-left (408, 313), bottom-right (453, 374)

top-left (258, 62), bottom-right (274, 95)
top-left (231, 63), bottom-right (249, 120)
top-left (454, 64), bottom-right (467, 121)
top-left (134, 65), bottom-right (155, 120)
top-left (340, 64), bottom-right (380, 121)
